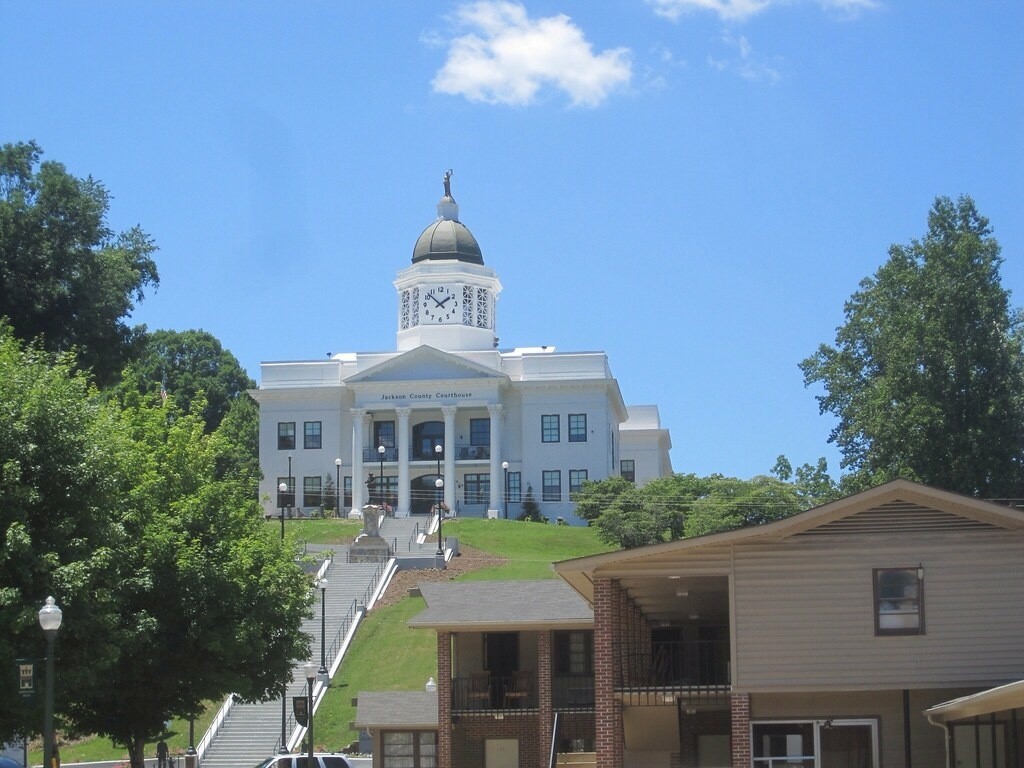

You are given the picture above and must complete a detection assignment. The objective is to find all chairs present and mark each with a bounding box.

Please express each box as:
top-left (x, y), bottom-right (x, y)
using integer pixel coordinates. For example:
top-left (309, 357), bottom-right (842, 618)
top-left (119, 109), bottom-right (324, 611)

top-left (468, 669), bottom-right (530, 708)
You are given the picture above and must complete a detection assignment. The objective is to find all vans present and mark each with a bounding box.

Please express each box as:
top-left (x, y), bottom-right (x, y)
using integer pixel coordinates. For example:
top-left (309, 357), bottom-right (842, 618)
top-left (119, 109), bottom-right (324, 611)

top-left (254, 751), bottom-right (353, 768)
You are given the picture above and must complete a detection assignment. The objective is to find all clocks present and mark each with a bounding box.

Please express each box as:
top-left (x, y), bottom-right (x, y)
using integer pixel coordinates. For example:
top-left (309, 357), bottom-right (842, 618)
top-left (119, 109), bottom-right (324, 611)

top-left (418, 284), bottom-right (463, 324)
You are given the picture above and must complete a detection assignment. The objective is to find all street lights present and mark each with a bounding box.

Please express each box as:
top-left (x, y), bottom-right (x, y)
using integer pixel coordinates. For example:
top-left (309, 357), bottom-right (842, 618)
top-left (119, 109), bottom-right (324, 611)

top-left (335, 459), bottom-right (342, 518)
top-left (502, 462), bottom-right (509, 519)
top-left (436, 478), bottom-right (444, 555)
top-left (302, 659), bottom-right (316, 768)
top-left (318, 579), bottom-right (328, 673)
top-left (279, 483), bottom-right (287, 546)
top-left (435, 445), bottom-right (443, 509)
top-left (39, 595), bottom-right (63, 768)
top-left (378, 446), bottom-right (386, 511)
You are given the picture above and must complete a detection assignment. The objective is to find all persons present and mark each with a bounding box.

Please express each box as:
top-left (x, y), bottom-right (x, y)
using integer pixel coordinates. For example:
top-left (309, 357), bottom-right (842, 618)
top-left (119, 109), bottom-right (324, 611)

top-left (167, 757), bottom-right (174, 768)
top-left (443, 168), bottom-right (453, 196)
top-left (157, 739), bottom-right (169, 768)
top-left (301, 739), bottom-right (307, 752)
